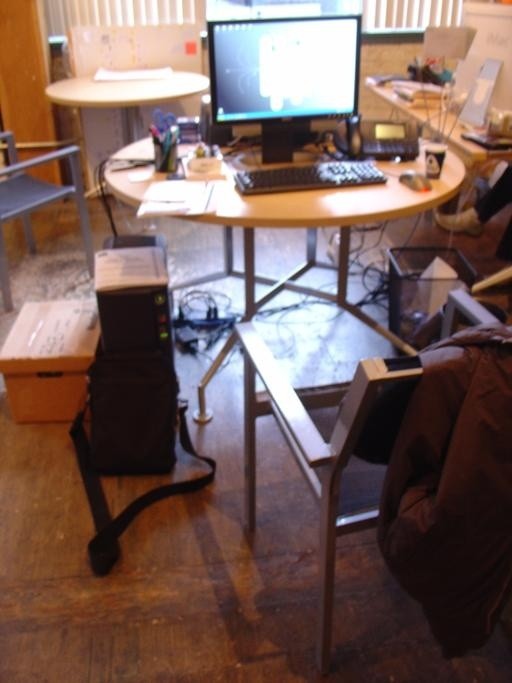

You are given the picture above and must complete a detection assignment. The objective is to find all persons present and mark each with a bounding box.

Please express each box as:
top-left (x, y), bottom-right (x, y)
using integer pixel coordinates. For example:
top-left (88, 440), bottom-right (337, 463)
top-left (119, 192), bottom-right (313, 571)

top-left (435, 163), bottom-right (511, 271)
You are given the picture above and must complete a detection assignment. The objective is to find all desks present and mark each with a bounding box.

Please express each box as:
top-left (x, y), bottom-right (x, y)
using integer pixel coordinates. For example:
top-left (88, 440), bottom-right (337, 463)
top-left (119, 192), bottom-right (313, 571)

top-left (365, 77), bottom-right (512, 163)
top-left (103, 119), bottom-right (470, 423)
top-left (45, 70), bottom-right (210, 199)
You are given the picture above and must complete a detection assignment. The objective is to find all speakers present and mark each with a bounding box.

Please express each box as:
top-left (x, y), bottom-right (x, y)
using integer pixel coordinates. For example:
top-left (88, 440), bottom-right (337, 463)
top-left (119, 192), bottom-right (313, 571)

top-left (199, 93), bottom-right (232, 147)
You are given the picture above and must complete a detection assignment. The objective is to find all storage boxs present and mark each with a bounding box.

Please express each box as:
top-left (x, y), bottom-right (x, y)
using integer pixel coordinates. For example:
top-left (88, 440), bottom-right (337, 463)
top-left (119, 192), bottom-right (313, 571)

top-left (0, 298), bottom-right (102, 424)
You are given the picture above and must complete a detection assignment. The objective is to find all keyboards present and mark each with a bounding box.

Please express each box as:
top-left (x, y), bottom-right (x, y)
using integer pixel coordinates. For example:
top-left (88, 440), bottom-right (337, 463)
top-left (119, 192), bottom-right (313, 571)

top-left (235, 159), bottom-right (389, 195)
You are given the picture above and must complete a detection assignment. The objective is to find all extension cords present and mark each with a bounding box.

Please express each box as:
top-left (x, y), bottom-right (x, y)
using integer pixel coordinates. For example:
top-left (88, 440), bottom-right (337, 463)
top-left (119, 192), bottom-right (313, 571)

top-left (179, 314), bottom-right (234, 328)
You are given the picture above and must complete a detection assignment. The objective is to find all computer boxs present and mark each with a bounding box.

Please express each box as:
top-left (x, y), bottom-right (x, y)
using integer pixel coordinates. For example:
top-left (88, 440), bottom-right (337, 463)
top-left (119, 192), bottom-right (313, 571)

top-left (91, 240), bottom-right (173, 352)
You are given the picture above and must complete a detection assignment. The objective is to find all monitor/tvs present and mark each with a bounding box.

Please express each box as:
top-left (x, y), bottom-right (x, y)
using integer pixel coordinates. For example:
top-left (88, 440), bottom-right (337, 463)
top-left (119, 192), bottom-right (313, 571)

top-left (205, 13), bottom-right (363, 145)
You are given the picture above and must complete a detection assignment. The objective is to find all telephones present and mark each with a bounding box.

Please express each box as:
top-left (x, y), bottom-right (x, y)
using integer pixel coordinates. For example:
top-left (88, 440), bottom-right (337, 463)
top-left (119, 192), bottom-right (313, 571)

top-left (316, 114), bottom-right (419, 159)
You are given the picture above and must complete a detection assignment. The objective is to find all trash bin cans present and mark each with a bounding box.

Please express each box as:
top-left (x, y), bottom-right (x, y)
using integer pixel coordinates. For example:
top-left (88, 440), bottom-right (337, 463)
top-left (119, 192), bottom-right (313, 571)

top-left (387, 245), bottom-right (478, 357)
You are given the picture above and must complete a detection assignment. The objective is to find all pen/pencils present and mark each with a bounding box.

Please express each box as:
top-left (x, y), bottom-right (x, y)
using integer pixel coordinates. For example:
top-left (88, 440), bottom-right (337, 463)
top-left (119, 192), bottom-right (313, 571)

top-left (149, 124), bottom-right (179, 172)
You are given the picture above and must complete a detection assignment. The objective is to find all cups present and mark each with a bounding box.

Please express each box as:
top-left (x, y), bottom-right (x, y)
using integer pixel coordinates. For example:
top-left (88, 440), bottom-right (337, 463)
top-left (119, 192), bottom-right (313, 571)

top-left (424, 144), bottom-right (444, 179)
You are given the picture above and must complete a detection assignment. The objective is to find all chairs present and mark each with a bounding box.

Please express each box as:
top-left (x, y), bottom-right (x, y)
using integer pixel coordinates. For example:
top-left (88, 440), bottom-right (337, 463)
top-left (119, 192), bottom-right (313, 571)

top-left (0, 130), bottom-right (94, 313)
top-left (234, 289), bottom-right (511, 677)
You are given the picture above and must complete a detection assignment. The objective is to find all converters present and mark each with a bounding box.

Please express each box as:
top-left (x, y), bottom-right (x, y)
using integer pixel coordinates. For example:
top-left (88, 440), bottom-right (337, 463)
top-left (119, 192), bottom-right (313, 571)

top-left (176, 326), bottom-right (198, 352)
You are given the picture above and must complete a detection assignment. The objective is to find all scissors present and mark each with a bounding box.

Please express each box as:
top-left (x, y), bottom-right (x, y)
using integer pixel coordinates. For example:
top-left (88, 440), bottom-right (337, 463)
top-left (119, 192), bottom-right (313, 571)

top-left (152, 109), bottom-right (175, 132)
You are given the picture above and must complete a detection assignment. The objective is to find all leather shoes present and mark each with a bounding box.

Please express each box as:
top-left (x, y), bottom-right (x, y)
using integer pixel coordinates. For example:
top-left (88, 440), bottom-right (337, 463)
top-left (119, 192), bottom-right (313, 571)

top-left (435, 207), bottom-right (483, 238)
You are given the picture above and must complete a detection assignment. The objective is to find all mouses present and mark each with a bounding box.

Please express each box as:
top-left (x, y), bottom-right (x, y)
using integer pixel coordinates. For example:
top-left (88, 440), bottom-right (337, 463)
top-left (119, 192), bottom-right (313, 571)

top-left (399, 167), bottom-right (430, 193)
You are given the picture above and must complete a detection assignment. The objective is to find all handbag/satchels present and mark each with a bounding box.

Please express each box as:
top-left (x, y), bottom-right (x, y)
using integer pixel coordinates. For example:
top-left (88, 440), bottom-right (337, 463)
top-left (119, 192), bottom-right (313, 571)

top-left (85, 356), bottom-right (180, 477)
